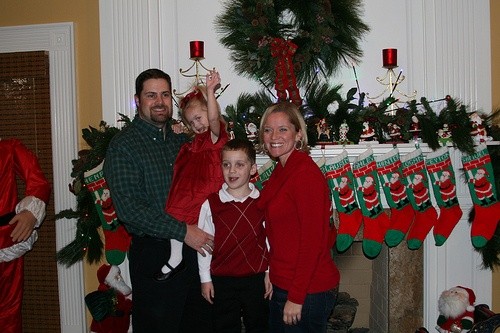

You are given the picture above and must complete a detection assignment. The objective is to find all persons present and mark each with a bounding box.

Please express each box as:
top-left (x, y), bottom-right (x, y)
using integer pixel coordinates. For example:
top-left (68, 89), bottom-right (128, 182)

top-left (106, 68), bottom-right (338, 333)
top-left (0, 137), bottom-right (50, 333)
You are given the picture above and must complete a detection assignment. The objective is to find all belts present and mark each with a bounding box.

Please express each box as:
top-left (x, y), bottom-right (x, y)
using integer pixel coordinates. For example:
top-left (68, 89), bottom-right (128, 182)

top-left (0, 212), bottom-right (16, 226)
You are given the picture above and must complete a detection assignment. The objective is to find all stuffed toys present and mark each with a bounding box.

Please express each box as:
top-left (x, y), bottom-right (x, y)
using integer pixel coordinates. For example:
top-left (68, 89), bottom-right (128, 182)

top-left (89, 264), bottom-right (133, 333)
top-left (435, 286), bottom-right (477, 333)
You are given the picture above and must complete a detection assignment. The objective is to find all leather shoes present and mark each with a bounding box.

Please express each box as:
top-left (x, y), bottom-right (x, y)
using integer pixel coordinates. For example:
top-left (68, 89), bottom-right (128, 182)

top-left (152, 259), bottom-right (184, 281)
